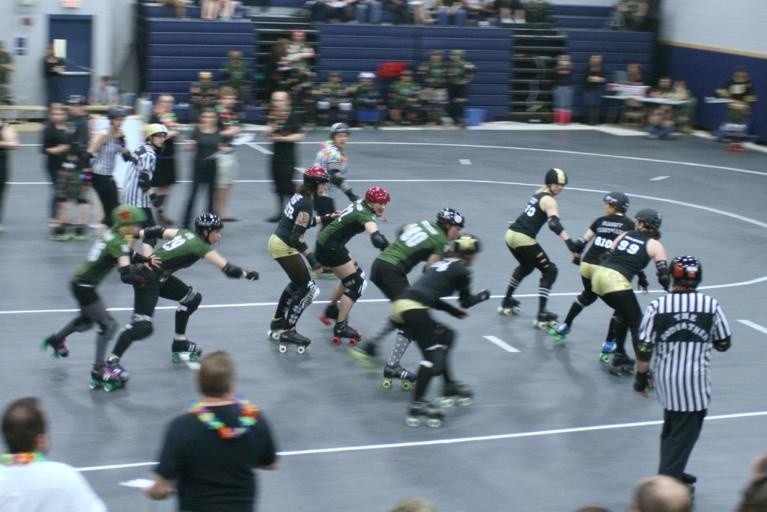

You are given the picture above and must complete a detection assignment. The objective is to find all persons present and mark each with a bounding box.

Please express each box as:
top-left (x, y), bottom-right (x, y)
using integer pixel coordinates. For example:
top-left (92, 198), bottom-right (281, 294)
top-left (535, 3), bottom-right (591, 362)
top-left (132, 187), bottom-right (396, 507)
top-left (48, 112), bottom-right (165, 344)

top-left (43, 97), bottom-right (123, 242)
top-left (311, 123), bottom-right (361, 205)
top-left (183, 108), bottom-right (219, 225)
top-left (637, 476), bottom-right (692, 511)
top-left (220, 49), bottom-right (254, 101)
top-left (387, 233), bottom-right (492, 428)
top-left (0, 121), bottom-right (20, 230)
top-left (621, 63), bottom-right (696, 133)
top-left (190, 70), bottom-right (221, 106)
top-left (273, 31), bottom-right (318, 90)
top-left (589, 209), bottom-right (670, 383)
top-left (150, 348), bottom-right (281, 512)
top-left (314, 189), bottom-right (388, 346)
top-left (501, 169), bottom-right (569, 328)
top-left (215, 86), bottom-right (240, 222)
top-left (265, 91), bottom-right (306, 223)
top-left (715, 65), bottom-right (757, 152)
top-left (330, 1), bottom-right (528, 26)
top-left (152, 95), bottom-right (176, 212)
top-left (634, 255), bottom-right (732, 488)
top-left (270, 169), bottom-right (332, 351)
top-left (349, 204), bottom-right (464, 390)
top-left (0, 397), bottom-right (107, 512)
top-left (109, 213), bottom-right (260, 382)
top-left (585, 53), bottom-right (610, 125)
top-left (47, 203), bottom-right (161, 392)
top-left (304, 49), bottom-right (476, 125)
top-left (43, 47), bottom-right (68, 102)
top-left (549, 55), bottom-right (579, 125)
top-left (0, 41), bottom-right (14, 109)
top-left (122, 122), bottom-right (169, 224)
top-left (554, 192), bottom-right (636, 357)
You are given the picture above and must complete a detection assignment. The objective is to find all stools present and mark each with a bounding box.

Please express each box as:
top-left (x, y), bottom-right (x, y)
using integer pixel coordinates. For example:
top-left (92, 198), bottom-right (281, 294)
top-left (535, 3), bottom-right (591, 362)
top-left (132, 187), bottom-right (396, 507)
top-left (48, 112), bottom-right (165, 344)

top-left (173, 103), bottom-right (196, 121)
top-left (197, 105), bottom-right (425, 124)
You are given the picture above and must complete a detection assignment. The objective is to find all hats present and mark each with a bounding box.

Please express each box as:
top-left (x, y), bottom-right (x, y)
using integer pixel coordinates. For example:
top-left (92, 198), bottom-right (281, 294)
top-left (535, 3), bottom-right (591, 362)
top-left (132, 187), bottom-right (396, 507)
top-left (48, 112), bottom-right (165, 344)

top-left (68, 95), bottom-right (87, 104)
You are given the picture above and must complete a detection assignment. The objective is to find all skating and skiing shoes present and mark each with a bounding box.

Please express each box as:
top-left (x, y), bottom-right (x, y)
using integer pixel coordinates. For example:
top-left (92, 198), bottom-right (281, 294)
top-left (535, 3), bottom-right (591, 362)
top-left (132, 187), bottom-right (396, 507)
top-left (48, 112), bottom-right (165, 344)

top-left (170, 340), bottom-right (202, 363)
top-left (609, 355), bottom-right (633, 376)
top-left (40, 335), bottom-right (69, 360)
top-left (88, 365), bottom-right (122, 392)
top-left (278, 330), bottom-right (311, 354)
top-left (548, 322), bottom-right (571, 342)
top-left (533, 311), bottom-right (559, 330)
top-left (405, 402), bottom-right (445, 429)
top-left (47, 226), bottom-right (71, 241)
top-left (319, 306), bottom-right (340, 325)
top-left (599, 341), bottom-right (618, 365)
top-left (331, 322), bottom-right (361, 347)
top-left (348, 340), bottom-right (382, 369)
top-left (267, 317), bottom-right (286, 339)
top-left (496, 298), bottom-right (521, 316)
top-left (436, 381), bottom-right (474, 406)
top-left (383, 361), bottom-right (417, 390)
top-left (104, 357), bottom-right (130, 383)
top-left (69, 223), bottom-right (87, 241)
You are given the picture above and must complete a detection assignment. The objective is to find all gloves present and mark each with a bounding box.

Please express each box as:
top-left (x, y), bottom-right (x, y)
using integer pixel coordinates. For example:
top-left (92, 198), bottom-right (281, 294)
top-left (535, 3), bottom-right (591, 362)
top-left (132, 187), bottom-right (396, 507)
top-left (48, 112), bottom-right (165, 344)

top-left (633, 370), bottom-right (653, 392)
top-left (478, 291), bottom-right (489, 301)
top-left (320, 211), bottom-right (337, 225)
top-left (572, 256), bottom-right (581, 266)
top-left (565, 237), bottom-right (577, 252)
top-left (246, 270), bottom-right (259, 281)
top-left (305, 251), bottom-right (322, 269)
top-left (637, 270), bottom-right (650, 290)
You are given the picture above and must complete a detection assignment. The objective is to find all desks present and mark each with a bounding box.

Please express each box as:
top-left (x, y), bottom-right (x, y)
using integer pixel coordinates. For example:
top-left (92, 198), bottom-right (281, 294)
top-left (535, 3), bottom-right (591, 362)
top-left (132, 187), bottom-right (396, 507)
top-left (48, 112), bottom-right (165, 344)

top-left (601, 94), bottom-right (692, 124)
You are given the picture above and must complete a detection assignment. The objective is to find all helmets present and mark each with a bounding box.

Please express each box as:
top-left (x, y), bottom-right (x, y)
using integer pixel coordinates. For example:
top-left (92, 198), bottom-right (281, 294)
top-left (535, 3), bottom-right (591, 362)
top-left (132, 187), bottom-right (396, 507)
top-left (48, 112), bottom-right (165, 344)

top-left (365, 186), bottom-right (391, 205)
top-left (452, 233), bottom-right (480, 254)
top-left (107, 106), bottom-right (130, 117)
top-left (327, 123), bottom-right (352, 137)
top-left (302, 165), bottom-right (329, 182)
top-left (111, 204), bottom-right (150, 226)
top-left (634, 208), bottom-right (662, 230)
top-left (436, 208), bottom-right (466, 228)
top-left (669, 255), bottom-right (702, 281)
top-left (145, 124), bottom-right (168, 140)
top-left (545, 167), bottom-right (568, 186)
top-left (195, 214), bottom-right (224, 232)
top-left (601, 192), bottom-right (629, 211)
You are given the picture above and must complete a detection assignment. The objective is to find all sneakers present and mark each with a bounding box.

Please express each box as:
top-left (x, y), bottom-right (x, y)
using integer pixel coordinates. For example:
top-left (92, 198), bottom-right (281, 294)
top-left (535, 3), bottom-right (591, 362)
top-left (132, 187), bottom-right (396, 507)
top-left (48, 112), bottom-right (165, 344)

top-left (680, 472), bottom-right (697, 484)
